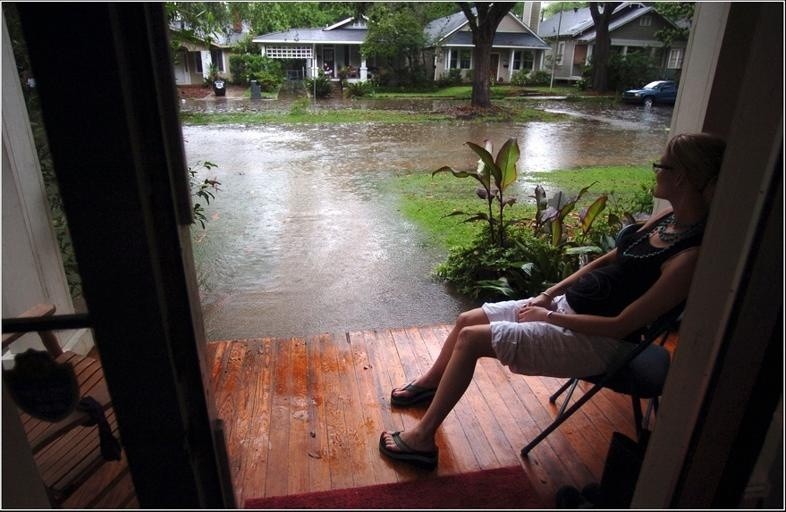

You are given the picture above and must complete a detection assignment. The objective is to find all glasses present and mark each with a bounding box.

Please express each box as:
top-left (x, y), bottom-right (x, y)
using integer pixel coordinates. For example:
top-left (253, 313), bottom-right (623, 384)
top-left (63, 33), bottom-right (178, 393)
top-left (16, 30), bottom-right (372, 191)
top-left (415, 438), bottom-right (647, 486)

top-left (653, 160), bottom-right (673, 172)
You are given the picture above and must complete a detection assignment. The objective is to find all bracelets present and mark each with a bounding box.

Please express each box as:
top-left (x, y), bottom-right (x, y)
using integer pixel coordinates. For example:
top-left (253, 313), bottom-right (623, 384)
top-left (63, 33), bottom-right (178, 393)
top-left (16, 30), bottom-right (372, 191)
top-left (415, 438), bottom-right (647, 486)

top-left (541, 292), bottom-right (553, 300)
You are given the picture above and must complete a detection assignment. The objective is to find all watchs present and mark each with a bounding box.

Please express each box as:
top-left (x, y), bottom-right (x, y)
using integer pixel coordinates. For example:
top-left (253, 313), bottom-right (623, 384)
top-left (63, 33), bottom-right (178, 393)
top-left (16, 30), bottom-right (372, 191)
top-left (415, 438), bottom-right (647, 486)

top-left (545, 311), bottom-right (553, 323)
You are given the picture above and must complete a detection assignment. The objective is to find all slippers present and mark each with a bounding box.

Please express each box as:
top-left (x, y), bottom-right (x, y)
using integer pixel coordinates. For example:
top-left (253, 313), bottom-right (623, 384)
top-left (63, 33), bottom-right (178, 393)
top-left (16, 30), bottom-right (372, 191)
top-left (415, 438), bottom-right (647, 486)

top-left (391, 378), bottom-right (436, 405)
top-left (379, 430), bottom-right (438, 463)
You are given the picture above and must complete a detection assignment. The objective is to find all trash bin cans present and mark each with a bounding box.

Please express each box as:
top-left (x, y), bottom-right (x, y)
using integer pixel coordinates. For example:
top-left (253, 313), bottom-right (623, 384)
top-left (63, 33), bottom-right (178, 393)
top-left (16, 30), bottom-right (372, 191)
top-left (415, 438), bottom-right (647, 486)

top-left (213, 80), bottom-right (226, 96)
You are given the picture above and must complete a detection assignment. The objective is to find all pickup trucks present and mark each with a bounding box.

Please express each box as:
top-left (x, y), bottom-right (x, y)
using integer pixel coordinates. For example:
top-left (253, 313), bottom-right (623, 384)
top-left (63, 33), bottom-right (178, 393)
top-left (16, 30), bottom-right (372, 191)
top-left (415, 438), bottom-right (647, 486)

top-left (622, 79), bottom-right (676, 106)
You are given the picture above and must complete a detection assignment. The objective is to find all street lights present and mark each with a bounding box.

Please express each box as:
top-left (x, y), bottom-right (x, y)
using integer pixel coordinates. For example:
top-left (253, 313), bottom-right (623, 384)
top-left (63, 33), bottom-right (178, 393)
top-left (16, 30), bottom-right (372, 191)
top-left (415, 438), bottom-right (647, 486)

top-left (550, 2), bottom-right (578, 91)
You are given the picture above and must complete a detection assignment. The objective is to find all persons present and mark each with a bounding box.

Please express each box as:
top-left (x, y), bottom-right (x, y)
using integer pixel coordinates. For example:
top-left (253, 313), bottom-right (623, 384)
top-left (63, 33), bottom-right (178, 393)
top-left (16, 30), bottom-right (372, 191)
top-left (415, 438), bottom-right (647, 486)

top-left (380, 135), bottom-right (725, 464)
top-left (323, 63), bottom-right (332, 79)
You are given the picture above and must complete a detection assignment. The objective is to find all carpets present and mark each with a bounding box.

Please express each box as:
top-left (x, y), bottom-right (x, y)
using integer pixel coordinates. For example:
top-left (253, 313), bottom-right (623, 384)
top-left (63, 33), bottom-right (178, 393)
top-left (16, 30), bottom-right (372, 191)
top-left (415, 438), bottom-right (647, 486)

top-left (238, 463), bottom-right (546, 509)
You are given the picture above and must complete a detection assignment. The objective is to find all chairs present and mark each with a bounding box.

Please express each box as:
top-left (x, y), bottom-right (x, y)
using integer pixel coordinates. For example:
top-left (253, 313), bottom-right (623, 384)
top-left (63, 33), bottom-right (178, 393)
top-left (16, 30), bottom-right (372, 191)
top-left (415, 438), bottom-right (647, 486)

top-left (518, 299), bottom-right (689, 458)
top-left (3, 303), bottom-right (141, 508)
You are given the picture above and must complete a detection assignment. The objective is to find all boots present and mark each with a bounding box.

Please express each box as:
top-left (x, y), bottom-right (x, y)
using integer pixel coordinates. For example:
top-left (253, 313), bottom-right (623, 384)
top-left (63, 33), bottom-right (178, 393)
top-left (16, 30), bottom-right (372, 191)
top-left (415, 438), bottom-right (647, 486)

top-left (554, 433), bottom-right (643, 509)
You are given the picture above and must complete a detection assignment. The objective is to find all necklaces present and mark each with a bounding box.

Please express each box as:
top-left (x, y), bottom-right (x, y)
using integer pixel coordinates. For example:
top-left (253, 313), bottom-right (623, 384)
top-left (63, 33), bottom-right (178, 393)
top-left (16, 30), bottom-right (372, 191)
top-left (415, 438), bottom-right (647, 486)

top-left (622, 214), bottom-right (708, 258)
